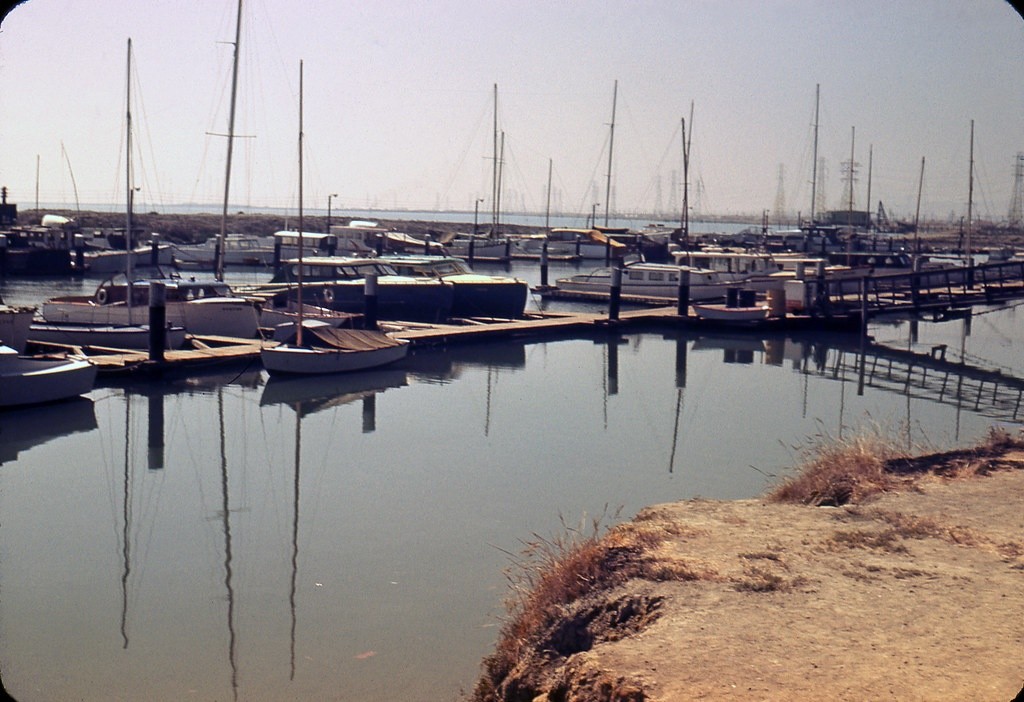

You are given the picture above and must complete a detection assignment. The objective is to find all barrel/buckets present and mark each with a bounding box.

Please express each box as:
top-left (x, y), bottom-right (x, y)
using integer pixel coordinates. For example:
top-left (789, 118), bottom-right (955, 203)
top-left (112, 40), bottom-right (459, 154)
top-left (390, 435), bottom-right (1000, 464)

top-left (737, 290), bottom-right (755, 308)
top-left (785, 281), bottom-right (804, 312)
top-left (727, 287), bottom-right (744, 308)
top-left (767, 288), bottom-right (786, 317)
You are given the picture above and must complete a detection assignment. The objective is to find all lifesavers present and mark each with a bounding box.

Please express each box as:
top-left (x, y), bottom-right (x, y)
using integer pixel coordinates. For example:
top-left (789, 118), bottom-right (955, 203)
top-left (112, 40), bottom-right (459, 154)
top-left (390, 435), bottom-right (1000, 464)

top-left (323, 288), bottom-right (336, 302)
top-left (97, 290), bottom-right (108, 305)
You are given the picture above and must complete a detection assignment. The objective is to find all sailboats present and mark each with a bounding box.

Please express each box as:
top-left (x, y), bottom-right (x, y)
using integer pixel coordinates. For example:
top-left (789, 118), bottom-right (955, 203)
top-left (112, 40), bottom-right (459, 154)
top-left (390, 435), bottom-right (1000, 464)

top-left (0, 0), bottom-right (1024, 408)
